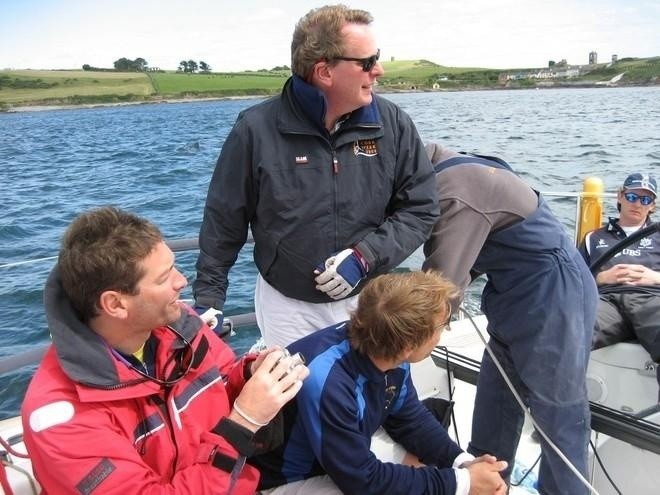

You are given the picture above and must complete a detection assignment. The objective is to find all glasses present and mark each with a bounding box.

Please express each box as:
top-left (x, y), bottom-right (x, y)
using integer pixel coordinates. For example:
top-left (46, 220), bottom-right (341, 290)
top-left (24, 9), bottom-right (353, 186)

top-left (430, 297), bottom-right (453, 332)
top-left (316, 47), bottom-right (382, 72)
top-left (622, 190), bottom-right (654, 206)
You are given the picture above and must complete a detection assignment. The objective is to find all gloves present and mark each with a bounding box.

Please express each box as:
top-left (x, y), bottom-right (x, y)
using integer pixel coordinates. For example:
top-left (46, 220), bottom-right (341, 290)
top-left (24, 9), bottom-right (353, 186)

top-left (194, 306), bottom-right (237, 337)
top-left (314, 248), bottom-right (370, 301)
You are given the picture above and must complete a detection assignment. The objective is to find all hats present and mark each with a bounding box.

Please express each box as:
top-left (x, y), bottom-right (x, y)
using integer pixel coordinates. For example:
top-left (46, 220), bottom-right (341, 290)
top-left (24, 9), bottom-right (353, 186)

top-left (622, 173), bottom-right (659, 200)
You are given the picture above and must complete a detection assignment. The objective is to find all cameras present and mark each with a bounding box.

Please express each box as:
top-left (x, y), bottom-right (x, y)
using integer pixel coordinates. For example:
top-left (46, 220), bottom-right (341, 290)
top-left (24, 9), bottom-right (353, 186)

top-left (271, 348), bottom-right (306, 393)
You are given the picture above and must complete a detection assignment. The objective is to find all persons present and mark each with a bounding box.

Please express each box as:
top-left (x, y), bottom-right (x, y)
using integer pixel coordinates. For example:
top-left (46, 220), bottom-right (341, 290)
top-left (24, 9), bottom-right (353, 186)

top-left (247, 266), bottom-right (510, 495)
top-left (416, 136), bottom-right (598, 494)
top-left (577, 172), bottom-right (660, 406)
top-left (19, 207), bottom-right (344, 495)
top-left (186, 5), bottom-right (441, 468)
